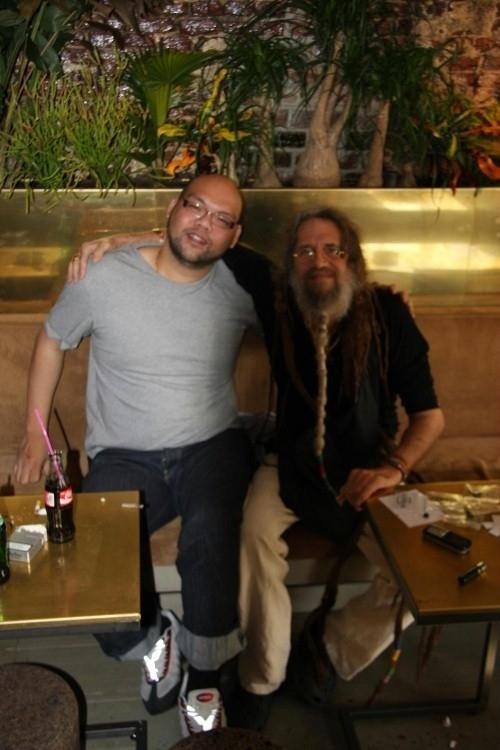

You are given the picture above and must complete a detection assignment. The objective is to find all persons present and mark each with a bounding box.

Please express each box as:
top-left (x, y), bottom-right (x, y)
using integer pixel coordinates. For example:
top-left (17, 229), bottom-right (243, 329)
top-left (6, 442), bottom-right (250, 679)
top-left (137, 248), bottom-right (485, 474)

top-left (64, 205), bottom-right (447, 733)
top-left (13, 166), bottom-right (422, 742)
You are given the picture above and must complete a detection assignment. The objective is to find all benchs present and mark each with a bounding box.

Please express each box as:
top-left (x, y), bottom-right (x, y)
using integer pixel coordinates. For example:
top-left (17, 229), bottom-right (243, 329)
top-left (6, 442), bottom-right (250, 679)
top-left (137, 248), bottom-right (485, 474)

top-left (1, 289), bottom-right (500, 559)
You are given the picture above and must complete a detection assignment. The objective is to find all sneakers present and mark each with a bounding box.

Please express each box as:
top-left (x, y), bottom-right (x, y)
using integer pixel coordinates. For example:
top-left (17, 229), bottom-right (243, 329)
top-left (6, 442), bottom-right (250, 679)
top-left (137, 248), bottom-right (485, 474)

top-left (140, 608), bottom-right (188, 716)
top-left (295, 614), bottom-right (337, 705)
top-left (227, 667), bottom-right (272, 732)
top-left (179, 669), bottom-right (227, 740)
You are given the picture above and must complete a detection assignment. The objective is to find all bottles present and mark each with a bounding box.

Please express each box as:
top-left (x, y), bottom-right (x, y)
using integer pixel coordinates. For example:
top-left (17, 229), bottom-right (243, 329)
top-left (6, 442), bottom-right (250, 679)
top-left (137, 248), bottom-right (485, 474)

top-left (44, 450), bottom-right (76, 543)
top-left (0, 515), bottom-right (11, 583)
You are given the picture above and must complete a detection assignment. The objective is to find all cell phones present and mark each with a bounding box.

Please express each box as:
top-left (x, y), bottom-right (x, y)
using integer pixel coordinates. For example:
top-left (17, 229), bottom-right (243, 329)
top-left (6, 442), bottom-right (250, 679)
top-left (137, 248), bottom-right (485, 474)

top-left (422, 523), bottom-right (473, 556)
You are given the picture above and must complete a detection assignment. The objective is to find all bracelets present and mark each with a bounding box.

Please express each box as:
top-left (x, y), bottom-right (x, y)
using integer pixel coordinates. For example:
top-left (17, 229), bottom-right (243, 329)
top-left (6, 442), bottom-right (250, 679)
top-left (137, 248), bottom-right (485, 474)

top-left (382, 459), bottom-right (409, 483)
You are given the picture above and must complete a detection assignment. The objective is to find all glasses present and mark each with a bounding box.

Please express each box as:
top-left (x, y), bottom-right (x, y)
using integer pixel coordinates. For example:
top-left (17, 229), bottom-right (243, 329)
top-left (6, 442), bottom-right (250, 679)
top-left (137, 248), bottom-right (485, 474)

top-left (183, 186), bottom-right (238, 229)
top-left (292, 246), bottom-right (346, 264)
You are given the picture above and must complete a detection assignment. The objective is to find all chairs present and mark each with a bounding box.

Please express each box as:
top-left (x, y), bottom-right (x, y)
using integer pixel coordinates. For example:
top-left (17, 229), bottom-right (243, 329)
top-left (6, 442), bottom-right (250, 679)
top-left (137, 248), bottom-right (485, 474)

top-left (1, 658), bottom-right (144, 750)
